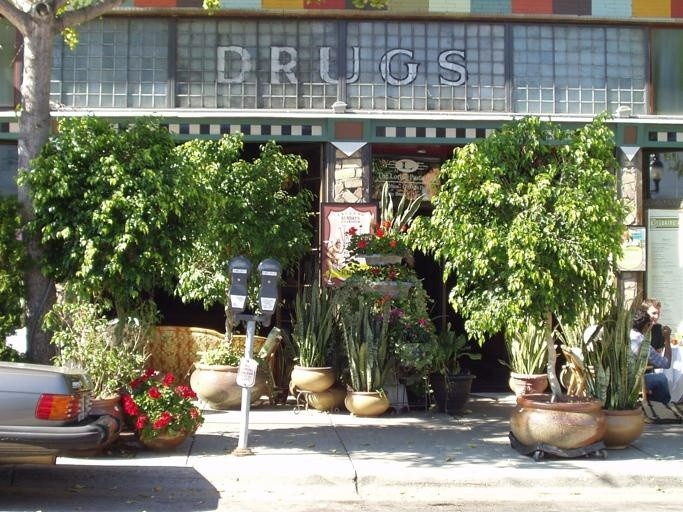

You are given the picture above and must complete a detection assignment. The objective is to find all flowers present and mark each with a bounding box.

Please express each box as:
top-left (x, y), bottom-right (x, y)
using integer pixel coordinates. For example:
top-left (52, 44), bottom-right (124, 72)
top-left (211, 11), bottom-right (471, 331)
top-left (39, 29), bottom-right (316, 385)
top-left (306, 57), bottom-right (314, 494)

top-left (354, 266), bottom-right (412, 282)
top-left (123, 368), bottom-right (205, 444)
top-left (345, 220), bottom-right (408, 255)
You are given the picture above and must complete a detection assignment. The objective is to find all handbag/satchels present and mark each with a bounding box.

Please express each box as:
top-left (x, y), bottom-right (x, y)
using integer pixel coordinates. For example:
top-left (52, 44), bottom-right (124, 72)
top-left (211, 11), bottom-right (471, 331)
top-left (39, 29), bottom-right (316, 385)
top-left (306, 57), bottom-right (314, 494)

top-left (643, 399), bottom-right (682, 423)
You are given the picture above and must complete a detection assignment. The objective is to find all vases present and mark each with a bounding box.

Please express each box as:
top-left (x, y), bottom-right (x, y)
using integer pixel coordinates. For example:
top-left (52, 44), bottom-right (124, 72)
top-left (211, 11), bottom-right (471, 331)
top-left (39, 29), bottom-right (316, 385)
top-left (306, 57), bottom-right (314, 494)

top-left (369, 281), bottom-right (411, 300)
top-left (137, 426), bottom-right (186, 451)
top-left (366, 253), bottom-right (404, 265)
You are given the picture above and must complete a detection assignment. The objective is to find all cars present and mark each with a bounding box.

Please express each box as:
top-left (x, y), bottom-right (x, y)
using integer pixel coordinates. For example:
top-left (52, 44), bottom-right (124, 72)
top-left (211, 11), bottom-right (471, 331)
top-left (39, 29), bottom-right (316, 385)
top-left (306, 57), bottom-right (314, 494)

top-left (0, 359), bottom-right (127, 467)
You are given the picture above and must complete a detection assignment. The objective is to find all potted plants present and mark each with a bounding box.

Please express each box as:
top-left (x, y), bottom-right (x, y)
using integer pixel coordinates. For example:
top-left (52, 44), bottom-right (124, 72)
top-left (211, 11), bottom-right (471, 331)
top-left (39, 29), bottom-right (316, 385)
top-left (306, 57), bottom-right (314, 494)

top-left (42, 299), bottom-right (162, 409)
top-left (190, 281), bottom-right (390, 417)
top-left (403, 112), bottom-right (653, 460)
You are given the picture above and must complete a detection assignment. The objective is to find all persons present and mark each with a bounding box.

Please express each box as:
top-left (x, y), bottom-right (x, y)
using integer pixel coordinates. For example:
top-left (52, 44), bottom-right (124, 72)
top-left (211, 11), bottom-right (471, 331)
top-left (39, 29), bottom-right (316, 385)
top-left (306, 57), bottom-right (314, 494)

top-left (324, 237), bottom-right (345, 286)
top-left (625, 310), bottom-right (672, 404)
top-left (635, 297), bottom-right (665, 353)
top-left (649, 155), bottom-right (664, 193)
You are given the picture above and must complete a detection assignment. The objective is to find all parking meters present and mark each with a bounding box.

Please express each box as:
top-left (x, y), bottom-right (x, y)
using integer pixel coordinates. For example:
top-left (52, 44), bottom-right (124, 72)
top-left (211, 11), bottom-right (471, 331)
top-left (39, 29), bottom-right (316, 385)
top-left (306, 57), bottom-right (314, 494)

top-left (222, 251), bottom-right (284, 460)
